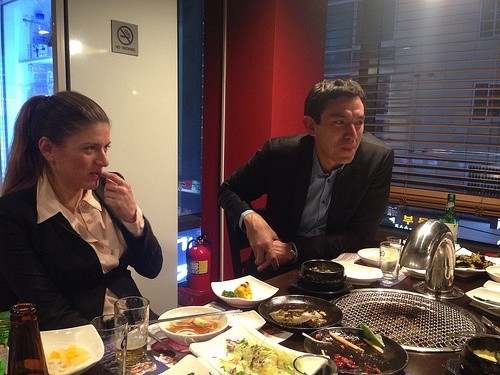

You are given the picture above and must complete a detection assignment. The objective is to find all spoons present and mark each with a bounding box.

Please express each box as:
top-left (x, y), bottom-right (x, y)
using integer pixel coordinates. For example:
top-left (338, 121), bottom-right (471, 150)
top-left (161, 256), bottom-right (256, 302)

top-left (147, 331), bottom-right (190, 363)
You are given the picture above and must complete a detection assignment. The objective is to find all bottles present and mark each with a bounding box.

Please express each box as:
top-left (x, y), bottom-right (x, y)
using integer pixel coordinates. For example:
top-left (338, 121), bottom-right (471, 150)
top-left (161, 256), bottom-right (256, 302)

top-left (0, 311), bottom-right (10, 375)
top-left (8, 303), bottom-right (49, 375)
top-left (440, 194), bottom-right (458, 244)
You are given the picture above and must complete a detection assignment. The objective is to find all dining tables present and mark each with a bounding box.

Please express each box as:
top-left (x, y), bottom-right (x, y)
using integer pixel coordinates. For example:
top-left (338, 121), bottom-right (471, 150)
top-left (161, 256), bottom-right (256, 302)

top-left (82, 241), bottom-right (500, 375)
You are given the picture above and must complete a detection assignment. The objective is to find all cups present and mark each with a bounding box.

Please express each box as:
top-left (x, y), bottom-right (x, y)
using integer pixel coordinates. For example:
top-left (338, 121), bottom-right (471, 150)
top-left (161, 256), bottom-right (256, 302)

top-left (293, 355), bottom-right (339, 375)
top-left (90, 313), bottom-right (129, 375)
top-left (114, 297), bottom-right (150, 366)
top-left (380, 235), bottom-right (403, 284)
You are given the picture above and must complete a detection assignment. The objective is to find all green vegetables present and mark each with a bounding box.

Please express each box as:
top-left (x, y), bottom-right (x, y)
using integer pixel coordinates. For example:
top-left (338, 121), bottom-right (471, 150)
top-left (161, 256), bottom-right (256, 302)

top-left (221, 290), bottom-right (237, 298)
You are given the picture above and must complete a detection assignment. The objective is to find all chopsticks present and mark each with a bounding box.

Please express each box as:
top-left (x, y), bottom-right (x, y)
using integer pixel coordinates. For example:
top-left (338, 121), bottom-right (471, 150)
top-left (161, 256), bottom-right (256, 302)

top-left (134, 309), bottom-right (243, 325)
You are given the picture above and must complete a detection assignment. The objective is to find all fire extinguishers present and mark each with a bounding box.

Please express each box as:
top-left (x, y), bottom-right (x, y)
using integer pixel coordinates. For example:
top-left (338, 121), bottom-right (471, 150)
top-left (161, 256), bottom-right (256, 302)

top-left (186, 233), bottom-right (212, 290)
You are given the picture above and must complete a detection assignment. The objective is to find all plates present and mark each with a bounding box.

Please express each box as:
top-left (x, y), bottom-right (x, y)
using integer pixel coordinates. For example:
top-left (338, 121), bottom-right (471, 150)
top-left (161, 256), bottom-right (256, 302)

top-left (258, 294), bottom-right (344, 336)
top-left (40, 324), bottom-right (105, 375)
top-left (290, 279), bottom-right (353, 300)
top-left (210, 275), bottom-right (280, 308)
top-left (303, 326), bottom-right (409, 375)
top-left (465, 281), bottom-right (500, 309)
top-left (332, 261), bottom-right (384, 286)
top-left (455, 247), bottom-right (500, 278)
top-left (402, 239), bottom-right (460, 278)
top-left (157, 311), bottom-right (330, 375)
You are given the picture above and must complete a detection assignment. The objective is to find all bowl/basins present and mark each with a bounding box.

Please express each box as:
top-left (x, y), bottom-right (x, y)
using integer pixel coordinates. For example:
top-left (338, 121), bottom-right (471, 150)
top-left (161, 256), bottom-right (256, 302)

top-left (357, 248), bottom-right (400, 270)
top-left (157, 306), bottom-right (230, 347)
top-left (460, 333), bottom-right (500, 375)
top-left (486, 265), bottom-right (500, 283)
top-left (298, 260), bottom-right (345, 292)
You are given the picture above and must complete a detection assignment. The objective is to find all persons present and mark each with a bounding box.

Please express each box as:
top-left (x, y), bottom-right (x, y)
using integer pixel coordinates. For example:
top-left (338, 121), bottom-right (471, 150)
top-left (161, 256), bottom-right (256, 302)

top-left (216, 77), bottom-right (395, 282)
top-left (0, 91), bottom-right (163, 332)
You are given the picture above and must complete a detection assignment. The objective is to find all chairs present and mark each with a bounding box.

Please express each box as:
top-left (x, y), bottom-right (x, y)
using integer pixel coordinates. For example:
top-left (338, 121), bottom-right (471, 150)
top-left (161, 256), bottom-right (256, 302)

top-left (226, 206), bottom-right (268, 281)
